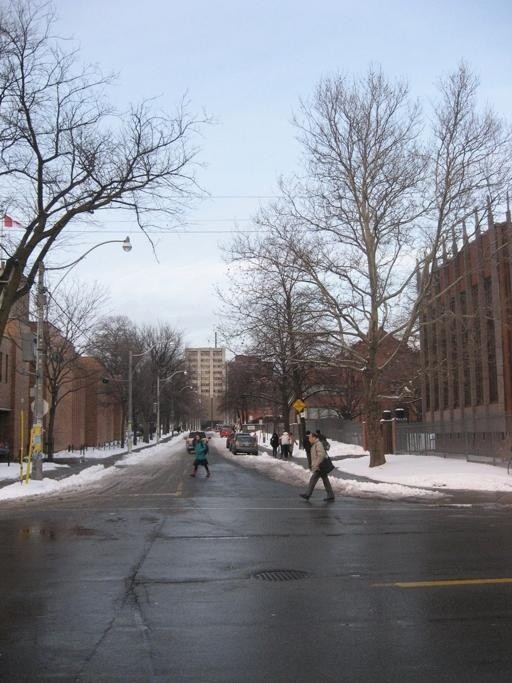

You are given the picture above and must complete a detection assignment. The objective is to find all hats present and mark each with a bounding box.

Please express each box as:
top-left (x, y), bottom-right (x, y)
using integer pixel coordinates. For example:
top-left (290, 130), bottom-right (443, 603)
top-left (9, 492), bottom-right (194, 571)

top-left (304, 431), bottom-right (319, 438)
top-left (283, 431), bottom-right (288, 435)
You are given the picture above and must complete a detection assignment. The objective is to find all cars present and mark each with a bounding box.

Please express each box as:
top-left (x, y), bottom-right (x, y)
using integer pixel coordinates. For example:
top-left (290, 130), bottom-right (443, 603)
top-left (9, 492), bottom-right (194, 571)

top-left (215, 423), bottom-right (258, 455)
top-left (183, 432), bottom-right (211, 454)
top-left (206, 427), bottom-right (211, 431)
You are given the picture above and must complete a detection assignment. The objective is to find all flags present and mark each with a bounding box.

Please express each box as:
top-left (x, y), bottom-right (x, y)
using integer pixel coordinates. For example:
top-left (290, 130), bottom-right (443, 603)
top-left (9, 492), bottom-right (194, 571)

top-left (3, 214), bottom-right (27, 229)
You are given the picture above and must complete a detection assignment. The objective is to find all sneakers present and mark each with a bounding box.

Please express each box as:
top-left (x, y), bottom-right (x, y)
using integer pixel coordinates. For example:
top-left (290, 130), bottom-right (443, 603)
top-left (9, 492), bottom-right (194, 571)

top-left (190, 473), bottom-right (211, 477)
top-left (298, 490), bottom-right (334, 501)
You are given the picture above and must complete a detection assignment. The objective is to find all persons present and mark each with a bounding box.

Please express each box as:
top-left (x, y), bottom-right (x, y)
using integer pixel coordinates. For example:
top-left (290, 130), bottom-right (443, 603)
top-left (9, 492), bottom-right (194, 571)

top-left (269, 428), bottom-right (327, 470)
top-left (190, 433), bottom-right (211, 477)
top-left (298, 432), bottom-right (335, 501)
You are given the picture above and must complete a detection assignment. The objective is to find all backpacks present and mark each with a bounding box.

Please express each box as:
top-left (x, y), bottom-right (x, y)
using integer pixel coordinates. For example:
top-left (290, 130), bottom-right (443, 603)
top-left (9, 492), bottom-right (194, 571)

top-left (192, 441), bottom-right (209, 454)
top-left (318, 459), bottom-right (335, 475)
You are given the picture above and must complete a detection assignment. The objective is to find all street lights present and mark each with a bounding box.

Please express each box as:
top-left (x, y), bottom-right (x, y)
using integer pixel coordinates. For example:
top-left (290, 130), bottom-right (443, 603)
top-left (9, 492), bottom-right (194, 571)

top-left (31, 235), bottom-right (132, 480)
top-left (127, 339), bottom-right (198, 456)
